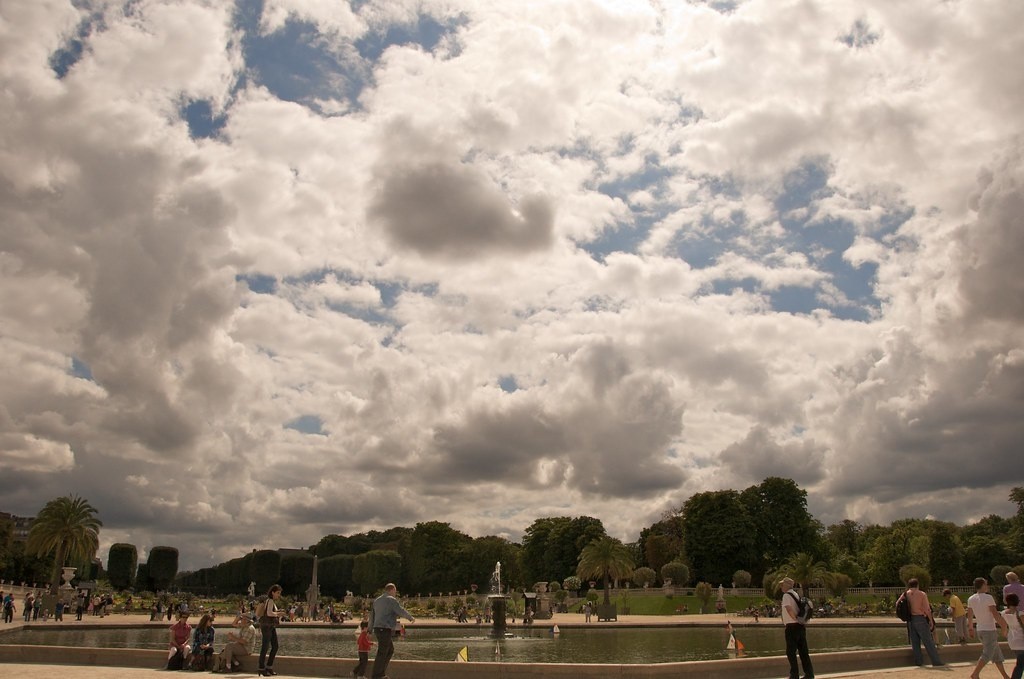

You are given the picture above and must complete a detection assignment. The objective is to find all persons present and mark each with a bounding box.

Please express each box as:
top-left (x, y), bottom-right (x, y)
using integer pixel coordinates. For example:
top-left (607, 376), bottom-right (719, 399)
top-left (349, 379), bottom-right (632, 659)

top-left (578, 601), bottom-right (598, 623)
top-left (350, 621), bottom-right (378, 679)
top-left (164, 610), bottom-right (192, 670)
top-left (1000, 594), bottom-right (1024, 679)
top-left (943, 589), bottom-right (968, 644)
top-left (1003, 572), bottom-right (1024, 610)
top-left (242, 600), bottom-right (353, 622)
top-left (219, 614), bottom-right (255, 673)
top-left (886, 601), bottom-right (967, 619)
top-left (0, 590), bottom-right (134, 624)
top-left (717, 584), bottom-right (725, 601)
top-left (140, 595), bottom-right (217, 621)
top-left (683, 605), bottom-right (688, 613)
top-left (895, 578), bottom-right (946, 666)
top-left (746, 597), bottom-right (869, 623)
top-left (260, 584), bottom-right (286, 677)
top-left (548, 607), bottom-right (553, 619)
top-left (968, 577), bottom-right (1001, 679)
top-left (778, 577), bottom-right (815, 679)
top-left (369, 583), bottom-right (415, 679)
top-left (717, 604), bottom-right (727, 613)
top-left (189, 615), bottom-right (215, 671)
top-left (449, 606), bottom-right (535, 625)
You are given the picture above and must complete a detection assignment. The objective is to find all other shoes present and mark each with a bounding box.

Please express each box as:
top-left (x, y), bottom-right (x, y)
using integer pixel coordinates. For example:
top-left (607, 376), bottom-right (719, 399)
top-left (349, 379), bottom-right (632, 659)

top-left (232, 663), bottom-right (239, 673)
top-left (350, 671), bottom-right (355, 677)
top-left (933, 662), bottom-right (944, 666)
top-left (958, 640), bottom-right (967, 644)
top-left (217, 666), bottom-right (231, 673)
top-left (357, 676), bottom-right (367, 679)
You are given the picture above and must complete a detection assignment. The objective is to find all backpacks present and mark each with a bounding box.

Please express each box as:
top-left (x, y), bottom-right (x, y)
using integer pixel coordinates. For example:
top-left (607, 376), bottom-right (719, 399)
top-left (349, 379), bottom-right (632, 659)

top-left (167, 649), bottom-right (183, 671)
top-left (785, 592), bottom-right (814, 625)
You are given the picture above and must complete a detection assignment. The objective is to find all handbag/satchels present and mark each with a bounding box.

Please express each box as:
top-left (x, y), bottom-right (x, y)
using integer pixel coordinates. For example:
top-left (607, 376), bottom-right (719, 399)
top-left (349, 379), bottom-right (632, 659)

top-left (192, 652), bottom-right (205, 671)
top-left (897, 590), bottom-right (912, 622)
top-left (212, 649), bottom-right (226, 673)
top-left (260, 598), bottom-right (279, 627)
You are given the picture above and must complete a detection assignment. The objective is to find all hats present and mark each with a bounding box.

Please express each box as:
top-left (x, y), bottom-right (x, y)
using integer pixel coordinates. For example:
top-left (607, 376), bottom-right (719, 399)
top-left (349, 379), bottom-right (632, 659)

top-left (779, 577), bottom-right (795, 587)
top-left (240, 613), bottom-right (254, 624)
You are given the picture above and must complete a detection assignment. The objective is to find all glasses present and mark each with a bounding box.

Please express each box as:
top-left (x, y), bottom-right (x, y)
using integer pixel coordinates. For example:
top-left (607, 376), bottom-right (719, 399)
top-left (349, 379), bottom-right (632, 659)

top-left (181, 617), bottom-right (188, 618)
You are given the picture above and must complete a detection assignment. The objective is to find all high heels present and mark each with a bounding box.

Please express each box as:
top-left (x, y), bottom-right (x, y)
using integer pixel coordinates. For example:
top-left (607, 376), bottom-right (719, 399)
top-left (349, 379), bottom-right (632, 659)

top-left (266, 666), bottom-right (277, 675)
top-left (258, 668), bottom-right (271, 676)
